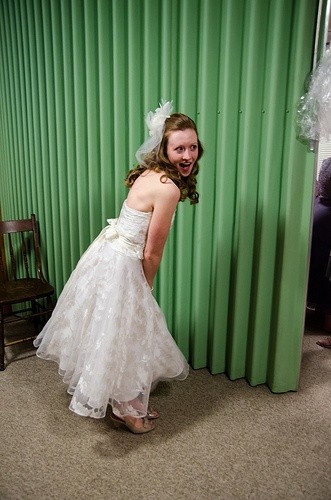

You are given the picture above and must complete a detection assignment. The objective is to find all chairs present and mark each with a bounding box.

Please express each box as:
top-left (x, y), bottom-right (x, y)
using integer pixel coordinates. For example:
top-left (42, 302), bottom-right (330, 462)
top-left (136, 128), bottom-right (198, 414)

top-left (0, 213), bottom-right (58, 372)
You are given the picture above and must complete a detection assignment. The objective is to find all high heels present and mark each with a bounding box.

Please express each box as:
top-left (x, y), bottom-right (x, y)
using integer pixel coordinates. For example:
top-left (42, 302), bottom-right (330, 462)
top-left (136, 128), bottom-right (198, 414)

top-left (107, 408), bottom-right (157, 434)
top-left (140, 407), bottom-right (160, 421)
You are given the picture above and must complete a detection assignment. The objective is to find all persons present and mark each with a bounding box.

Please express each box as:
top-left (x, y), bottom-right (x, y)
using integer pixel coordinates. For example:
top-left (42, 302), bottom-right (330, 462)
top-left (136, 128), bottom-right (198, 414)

top-left (31, 98), bottom-right (204, 434)
top-left (316, 338), bottom-right (331, 349)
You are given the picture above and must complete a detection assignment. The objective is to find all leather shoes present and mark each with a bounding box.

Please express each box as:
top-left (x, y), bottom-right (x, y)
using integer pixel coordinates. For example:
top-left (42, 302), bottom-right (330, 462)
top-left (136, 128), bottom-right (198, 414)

top-left (317, 335), bottom-right (331, 349)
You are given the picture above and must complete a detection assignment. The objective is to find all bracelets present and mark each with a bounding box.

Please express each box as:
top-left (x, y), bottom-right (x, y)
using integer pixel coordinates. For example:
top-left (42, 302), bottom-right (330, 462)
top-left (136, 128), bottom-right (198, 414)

top-left (151, 287), bottom-right (153, 290)
